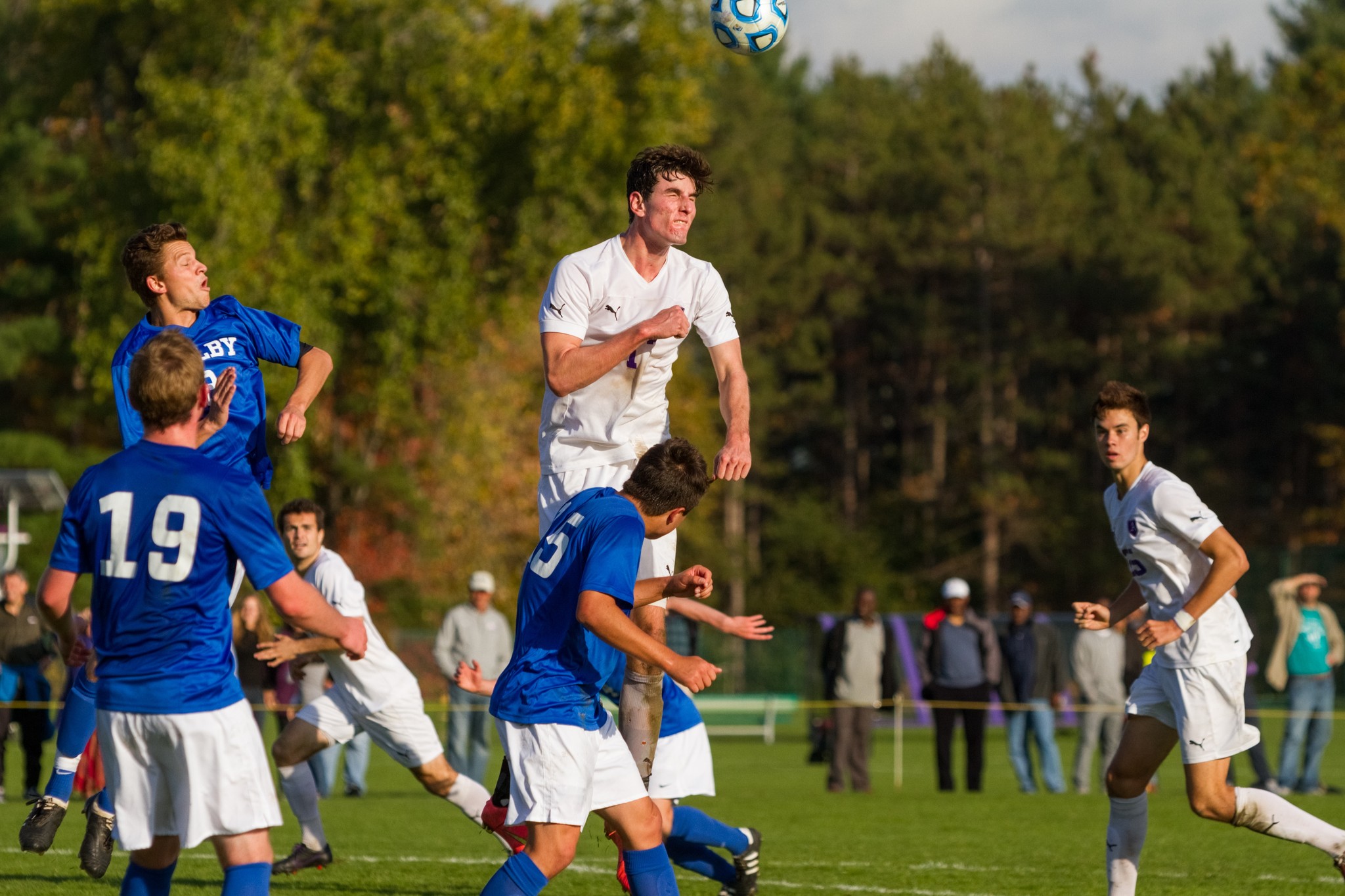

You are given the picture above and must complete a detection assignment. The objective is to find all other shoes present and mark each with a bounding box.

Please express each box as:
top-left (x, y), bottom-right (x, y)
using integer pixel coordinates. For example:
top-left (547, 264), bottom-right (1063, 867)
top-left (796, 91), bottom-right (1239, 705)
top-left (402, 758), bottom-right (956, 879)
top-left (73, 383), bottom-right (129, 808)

top-left (348, 786), bottom-right (360, 796)
top-left (1308, 786), bottom-right (1326, 797)
top-left (1262, 777), bottom-right (1290, 796)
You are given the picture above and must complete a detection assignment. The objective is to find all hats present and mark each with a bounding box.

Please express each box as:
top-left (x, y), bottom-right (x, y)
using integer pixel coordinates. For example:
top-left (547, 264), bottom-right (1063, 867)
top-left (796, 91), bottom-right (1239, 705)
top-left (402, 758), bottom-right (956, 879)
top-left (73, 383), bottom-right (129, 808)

top-left (1012, 591), bottom-right (1030, 609)
top-left (469, 570), bottom-right (497, 594)
top-left (941, 577), bottom-right (969, 598)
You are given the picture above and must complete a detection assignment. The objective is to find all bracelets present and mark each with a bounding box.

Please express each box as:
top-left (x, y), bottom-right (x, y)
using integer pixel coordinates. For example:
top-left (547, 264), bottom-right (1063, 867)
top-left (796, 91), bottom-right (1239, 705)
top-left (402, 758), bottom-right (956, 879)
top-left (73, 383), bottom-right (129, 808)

top-left (1172, 610), bottom-right (1197, 631)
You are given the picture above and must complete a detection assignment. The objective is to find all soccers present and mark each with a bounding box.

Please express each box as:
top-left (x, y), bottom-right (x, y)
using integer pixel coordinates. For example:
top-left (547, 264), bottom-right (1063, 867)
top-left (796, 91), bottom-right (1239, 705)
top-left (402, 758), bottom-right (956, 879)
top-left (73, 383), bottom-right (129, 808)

top-left (708, 0), bottom-right (790, 56)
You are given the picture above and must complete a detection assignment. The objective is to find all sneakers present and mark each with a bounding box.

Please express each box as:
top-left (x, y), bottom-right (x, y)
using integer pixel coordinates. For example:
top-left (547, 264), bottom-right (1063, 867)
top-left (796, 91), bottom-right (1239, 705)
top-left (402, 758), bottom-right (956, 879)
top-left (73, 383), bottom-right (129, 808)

top-left (718, 882), bottom-right (758, 896)
top-left (77, 792), bottom-right (115, 880)
top-left (1334, 850), bottom-right (1345, 880)
top-left (481, 798), bottom-right (529, 857)
top-left (603, 819), bottom-right (632, 896)
top-left (19, 792), bottom-right (68, 856)
top-left (732, 824), bottom-right (761, 894)
top-left (271, 843), bottom-right (333, 876)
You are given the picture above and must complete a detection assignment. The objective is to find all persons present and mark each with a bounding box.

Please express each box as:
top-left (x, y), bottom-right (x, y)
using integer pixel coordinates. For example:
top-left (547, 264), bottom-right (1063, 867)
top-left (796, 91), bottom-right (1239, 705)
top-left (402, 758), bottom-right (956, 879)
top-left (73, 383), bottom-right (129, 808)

top-left (481, 147), bottom-right (751, 890)
top-left (0, 333), bottom-right (1345, 896)
top-left (18, 222), bottom-right (333, 879)
